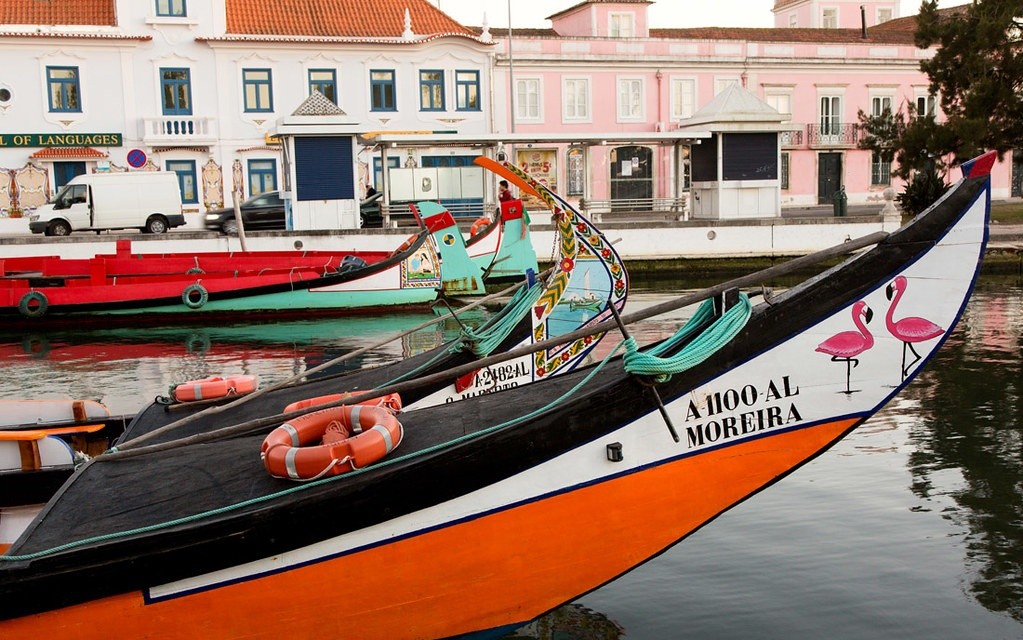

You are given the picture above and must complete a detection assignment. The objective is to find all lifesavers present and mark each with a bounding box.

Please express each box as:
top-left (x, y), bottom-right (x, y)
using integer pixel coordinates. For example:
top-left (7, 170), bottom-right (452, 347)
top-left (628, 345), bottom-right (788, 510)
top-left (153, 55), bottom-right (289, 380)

top-left (181, 283), bottom-right (209, 310)
top-left (470, 218), bottom-right (492, 238)
top-left (186, 333), bottom-right (211, 354)
top-left (23, 334), bottom-right (50, 358)
top-left (260, 404), bottom-right (402, 481)
top-left (18, 291), bottom-right (48, 320)
top-left (283, 389), bottom-right (402, 425)
top-left (186, 267), bottom-right (205, 276)
top-left (390, 233), bottom-right (420, 258)
top-left (174, 375), bottom-right (257, 401)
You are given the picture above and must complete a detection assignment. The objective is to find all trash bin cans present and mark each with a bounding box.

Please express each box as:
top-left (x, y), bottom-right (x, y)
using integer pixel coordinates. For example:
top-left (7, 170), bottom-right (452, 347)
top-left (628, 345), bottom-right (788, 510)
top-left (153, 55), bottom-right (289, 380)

top-left (833, 190), bottom-right (848, 217)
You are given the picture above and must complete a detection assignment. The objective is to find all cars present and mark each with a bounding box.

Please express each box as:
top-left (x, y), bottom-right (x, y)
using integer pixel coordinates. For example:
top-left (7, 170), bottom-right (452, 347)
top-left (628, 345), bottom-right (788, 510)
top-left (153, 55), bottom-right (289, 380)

top-left (203, 191), bottom-right (286, 235)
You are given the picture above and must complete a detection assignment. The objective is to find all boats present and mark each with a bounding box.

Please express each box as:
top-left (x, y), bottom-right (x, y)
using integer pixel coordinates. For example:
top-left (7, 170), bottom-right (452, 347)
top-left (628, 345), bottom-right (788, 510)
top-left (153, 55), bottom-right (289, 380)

top-left (0, 146), bottom-right (1000, 640)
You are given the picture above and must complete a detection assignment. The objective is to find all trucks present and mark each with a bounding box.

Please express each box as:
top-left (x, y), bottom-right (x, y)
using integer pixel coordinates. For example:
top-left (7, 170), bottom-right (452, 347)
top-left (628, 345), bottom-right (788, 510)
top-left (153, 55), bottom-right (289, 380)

top-left (28, 170), bottom-right (187, 235)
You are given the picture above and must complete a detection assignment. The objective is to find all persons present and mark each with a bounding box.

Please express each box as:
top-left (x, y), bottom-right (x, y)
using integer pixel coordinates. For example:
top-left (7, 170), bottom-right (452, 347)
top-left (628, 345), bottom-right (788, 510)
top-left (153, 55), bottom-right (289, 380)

top-left (365, 184), bottom-right (376, 199)
top-left (498, 180), bottom-right (513, 225)
